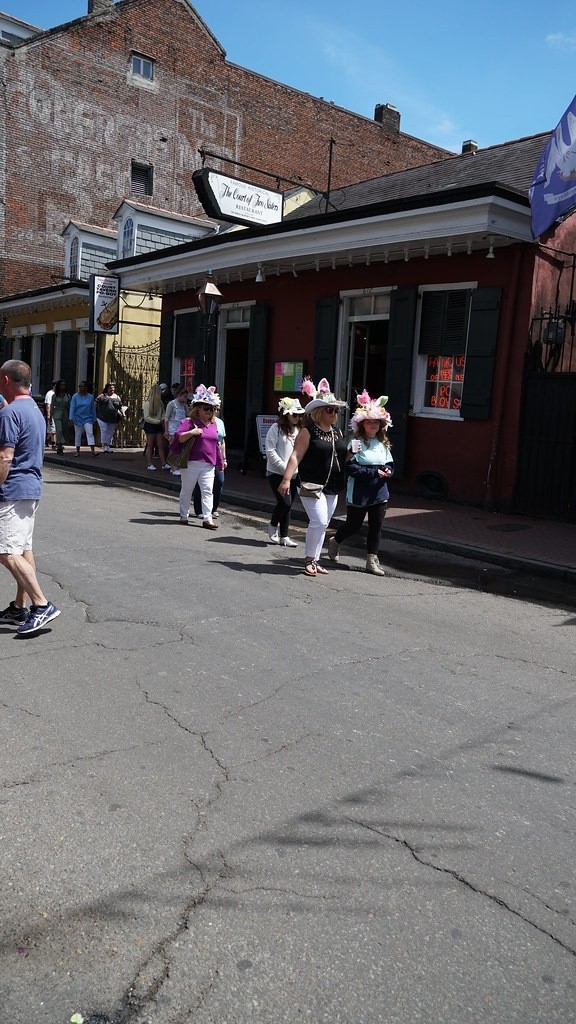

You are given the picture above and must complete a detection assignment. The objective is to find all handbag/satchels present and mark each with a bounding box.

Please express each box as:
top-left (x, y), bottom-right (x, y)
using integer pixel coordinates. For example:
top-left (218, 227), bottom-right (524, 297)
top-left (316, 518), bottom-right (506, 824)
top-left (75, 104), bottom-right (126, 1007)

top-left (298, 481), bottom-right (324, 498)
top-left (166, 416), bottom-right (199, 469)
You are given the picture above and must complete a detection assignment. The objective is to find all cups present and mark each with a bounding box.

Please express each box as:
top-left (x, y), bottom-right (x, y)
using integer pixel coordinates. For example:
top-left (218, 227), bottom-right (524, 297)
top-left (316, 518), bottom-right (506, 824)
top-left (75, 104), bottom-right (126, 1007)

top-left (352, 440), bottom-right (360, 453)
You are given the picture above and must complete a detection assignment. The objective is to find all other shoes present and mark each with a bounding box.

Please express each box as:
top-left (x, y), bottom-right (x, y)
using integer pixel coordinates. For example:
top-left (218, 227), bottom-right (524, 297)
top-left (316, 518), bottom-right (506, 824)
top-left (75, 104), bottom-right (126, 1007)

top-left (148, 464), bottom-right (182, 476)
top-left (45, 441), bottom-right (114, 457)
top-left (280, 536), bottom-right (298, 546)
top-left (268, 523), bottom-right (280, 542)
top-left (202, 521), bottom-right (218, 529)
top-left (304, 557), bottom-right (317, 575)
top-left (196, 513), bottom-right (204, 519)
top-left (180, 515), bottom-right (187, 524)
top-left (314, 560), bottom-right (330, 574)
top-left (213, 512), bottom-right (219, 517)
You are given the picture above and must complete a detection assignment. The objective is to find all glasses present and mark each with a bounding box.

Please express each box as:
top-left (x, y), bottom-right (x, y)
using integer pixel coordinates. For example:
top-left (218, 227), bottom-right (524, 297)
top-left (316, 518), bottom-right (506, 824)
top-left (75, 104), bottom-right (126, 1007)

top-left (78, 385), bottom-right (86, 388)
top-left (198, 405), bottom-right (214, 413)
top-left (288, 412), bottom-right (303, 418)
top-left (323, 407), bottom-right (339, 415)
top-left (182, 394), bottom-right (187, 398)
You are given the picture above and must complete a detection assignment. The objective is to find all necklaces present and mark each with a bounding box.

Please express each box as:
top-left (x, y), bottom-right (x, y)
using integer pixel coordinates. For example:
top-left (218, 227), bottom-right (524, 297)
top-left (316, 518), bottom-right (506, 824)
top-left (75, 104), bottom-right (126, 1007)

top-left (311, 423), bottom-right (343, 442)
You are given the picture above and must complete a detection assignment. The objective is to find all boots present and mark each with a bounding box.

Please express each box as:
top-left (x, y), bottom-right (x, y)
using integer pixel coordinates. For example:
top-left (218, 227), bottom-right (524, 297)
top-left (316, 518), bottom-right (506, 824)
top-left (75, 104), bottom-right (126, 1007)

top-left (327, 536), bottom-right (341, 561)
top-left (366, 553), bottom-right (386, 576)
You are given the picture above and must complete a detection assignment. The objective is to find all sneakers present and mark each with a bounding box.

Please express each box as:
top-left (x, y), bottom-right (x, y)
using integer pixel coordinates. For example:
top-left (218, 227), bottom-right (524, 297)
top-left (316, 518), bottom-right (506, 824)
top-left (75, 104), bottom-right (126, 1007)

top-left (15, 601), bottom-right (61, 635)
top-left (0, 601), bottom-right (29, 625)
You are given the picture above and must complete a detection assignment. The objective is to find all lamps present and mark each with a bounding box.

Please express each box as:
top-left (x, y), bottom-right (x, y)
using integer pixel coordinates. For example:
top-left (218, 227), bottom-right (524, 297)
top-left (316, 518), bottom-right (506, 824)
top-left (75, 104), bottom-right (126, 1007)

top-left (215, 277), bottom-right (219, 286)
top-left (225, 273), bottom-right (231, 283)
top-left (183, 282), bottom-right (186, 290)
top-left (193, 282), bottom-right (197, 289)
top-left (153, 287), bottom-right (158, 297)
top-left (276, 265), bottom-right (280, 276)
top-left (292, 263), bottom-right (298, 278)
top-left (366, 254), bottom-right (371, 265)
top-left (486, 238), bottom-right (496, 258)
top-left (147, 288), bottom-right (153, 300)
top-left (384, 251), bottom-right (390, 263)
top-left (239, 273), bottom-right (243, 282)
top-left (446, 242), bottom-right (452, 256)
top-left (332, 258), bottom-right (336, 270)
top-left (173, 283), bottom-right (177, 292)
top-left (315, 260), bottom-right (321, 271)
top-left (256, 263), bottom-right (264, 282)
top-left (466, 241), bottom-right (473, 254)
top-left (403, 248), bottom-right (410, 262)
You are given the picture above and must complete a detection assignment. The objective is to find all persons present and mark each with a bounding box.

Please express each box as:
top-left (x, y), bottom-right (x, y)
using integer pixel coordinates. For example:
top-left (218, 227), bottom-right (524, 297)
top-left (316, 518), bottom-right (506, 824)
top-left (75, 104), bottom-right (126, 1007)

top-left (265, 396), bottom-right (306, 547)
top-left (143, 383), bottom-right (191, 476)
top-left (0, 359), bottom-right (62, 636)
top-left (278, 394), bottom-right (351, 577)
top-left (328, 407), bottom-right (396, 576)
top-left (95, 382), bottom-right (128, 454)
top-left (174, 383), bottom-right (227, 529)
top-left (69, 381), bottom-right (99, 457)
top-left (44, 379), bottom-right (72, 455)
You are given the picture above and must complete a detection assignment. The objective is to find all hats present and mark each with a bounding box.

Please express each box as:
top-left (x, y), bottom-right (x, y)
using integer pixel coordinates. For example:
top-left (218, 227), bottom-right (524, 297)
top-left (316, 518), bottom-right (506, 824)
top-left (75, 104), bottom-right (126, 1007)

top-left (277, 397), bottom-right (306, 416)
top-left (302, 375), bottom-right (348, 416)
top-left (350, 389), bottom-right (393, 434)
top-left (159, 383), bottom-right (167, 390)
top-left (191, 385), bottom-right (221, 410)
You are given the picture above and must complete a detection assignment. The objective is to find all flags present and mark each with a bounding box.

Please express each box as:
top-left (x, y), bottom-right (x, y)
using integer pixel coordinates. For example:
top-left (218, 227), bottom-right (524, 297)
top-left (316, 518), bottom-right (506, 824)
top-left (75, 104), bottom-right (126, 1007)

top-left (528, 96), bottom-right (576, 244)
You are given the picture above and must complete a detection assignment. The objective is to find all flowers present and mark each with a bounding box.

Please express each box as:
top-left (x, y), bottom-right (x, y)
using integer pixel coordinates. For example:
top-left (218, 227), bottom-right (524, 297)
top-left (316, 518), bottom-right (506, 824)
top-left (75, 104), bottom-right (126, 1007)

top-left (316, 386), bottom-right (336, 403)
top-left (191, 386), bottom-right (222, 406)
top-left (348, 387), bottom-right (394, 433)
top-left (278, 397), bottom-right (298, 415)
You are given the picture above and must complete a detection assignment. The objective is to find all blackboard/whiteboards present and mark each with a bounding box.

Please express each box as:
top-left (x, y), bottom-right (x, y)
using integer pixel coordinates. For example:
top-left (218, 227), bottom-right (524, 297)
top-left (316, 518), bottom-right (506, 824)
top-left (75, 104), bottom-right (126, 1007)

top-left (32, 396), bottom-right (47, 422)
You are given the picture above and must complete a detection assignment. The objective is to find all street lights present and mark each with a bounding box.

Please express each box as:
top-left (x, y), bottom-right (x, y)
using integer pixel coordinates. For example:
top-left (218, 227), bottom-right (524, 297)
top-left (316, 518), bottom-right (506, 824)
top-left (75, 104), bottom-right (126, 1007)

top-left (194, 282), bottom-right (223, 389)
